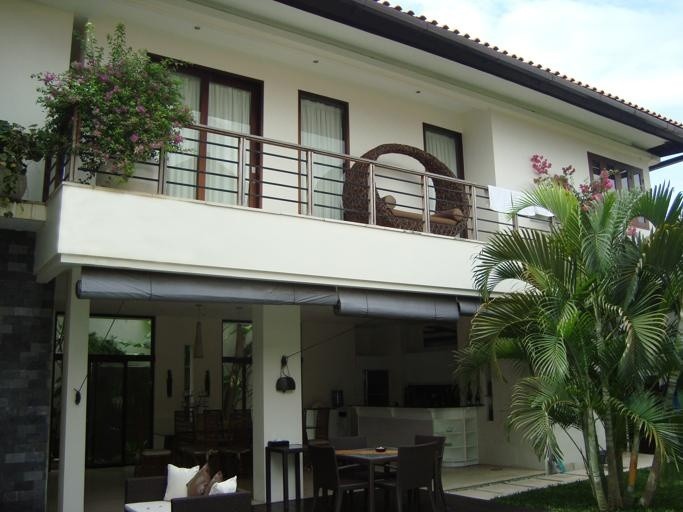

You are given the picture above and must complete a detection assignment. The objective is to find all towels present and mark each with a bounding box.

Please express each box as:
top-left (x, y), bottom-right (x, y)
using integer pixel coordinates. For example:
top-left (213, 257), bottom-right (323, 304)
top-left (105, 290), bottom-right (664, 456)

top-left (484, 184), bottom-right (512, 213)
top-left (534, 204), bottom-right (555, 216)
top-left (512, 192), bottom-right (535, 216)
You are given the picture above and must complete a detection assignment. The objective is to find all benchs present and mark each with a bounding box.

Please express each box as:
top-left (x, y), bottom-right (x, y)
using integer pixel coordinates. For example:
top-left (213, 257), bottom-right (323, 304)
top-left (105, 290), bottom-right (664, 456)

top-left (340, 143), bottom-right (469, 237)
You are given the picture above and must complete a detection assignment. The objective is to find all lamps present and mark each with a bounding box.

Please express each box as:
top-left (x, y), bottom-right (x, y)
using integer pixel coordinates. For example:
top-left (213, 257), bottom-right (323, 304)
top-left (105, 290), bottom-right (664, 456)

top-left (235, 305), bottom-right (244, 359)
top-left (275, 354), bottom-right (295, 392)
top-left (193, 300), bottom-right (203, 358)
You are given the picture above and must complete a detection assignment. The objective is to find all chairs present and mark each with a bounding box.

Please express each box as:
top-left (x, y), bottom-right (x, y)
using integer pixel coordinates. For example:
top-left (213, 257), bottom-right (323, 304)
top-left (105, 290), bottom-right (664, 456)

top-left (164, 407), bottom-right (252, 477)
top-left (264, 407), bottom-right (450, 512)
top-left (122, 468), bottom-right (250, 512)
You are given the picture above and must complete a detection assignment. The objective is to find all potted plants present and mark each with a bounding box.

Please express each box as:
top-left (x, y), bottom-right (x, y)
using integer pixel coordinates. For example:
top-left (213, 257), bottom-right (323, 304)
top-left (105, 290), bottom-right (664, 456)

top-left (0, 119), bottom-right (49, 205)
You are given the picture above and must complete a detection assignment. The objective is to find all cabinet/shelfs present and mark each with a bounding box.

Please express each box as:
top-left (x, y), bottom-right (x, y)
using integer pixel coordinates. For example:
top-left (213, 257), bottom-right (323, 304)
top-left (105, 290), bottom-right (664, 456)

top-left (356, 405), bottom-right (479, 466)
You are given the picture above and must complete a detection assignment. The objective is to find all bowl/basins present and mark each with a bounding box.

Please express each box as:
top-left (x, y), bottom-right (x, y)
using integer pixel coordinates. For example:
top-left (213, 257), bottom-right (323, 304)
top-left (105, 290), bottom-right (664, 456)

top-left (375, 447), bottom-right (387, 451)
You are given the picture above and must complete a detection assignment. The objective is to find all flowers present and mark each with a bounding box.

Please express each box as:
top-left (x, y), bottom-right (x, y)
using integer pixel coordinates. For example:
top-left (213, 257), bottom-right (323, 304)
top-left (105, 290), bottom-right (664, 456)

top-left (528, 151), bottom-right (639, 236)
top-left (30, 21), bottom-right (194, 183)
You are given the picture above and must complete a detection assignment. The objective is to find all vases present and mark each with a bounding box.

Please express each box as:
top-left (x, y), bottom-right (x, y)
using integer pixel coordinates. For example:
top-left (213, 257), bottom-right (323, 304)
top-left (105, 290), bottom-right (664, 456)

top-left (93, 156), bottom-right (124, 190)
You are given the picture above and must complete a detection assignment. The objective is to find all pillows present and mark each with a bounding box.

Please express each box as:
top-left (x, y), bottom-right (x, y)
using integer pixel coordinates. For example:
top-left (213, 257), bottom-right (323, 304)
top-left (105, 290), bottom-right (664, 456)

top-left (380, 196), bottom-right (396, 210)
top-left (161, 463), bottom-right (237, 500)
top-left (430, 207), bottom-right (463, 223)
